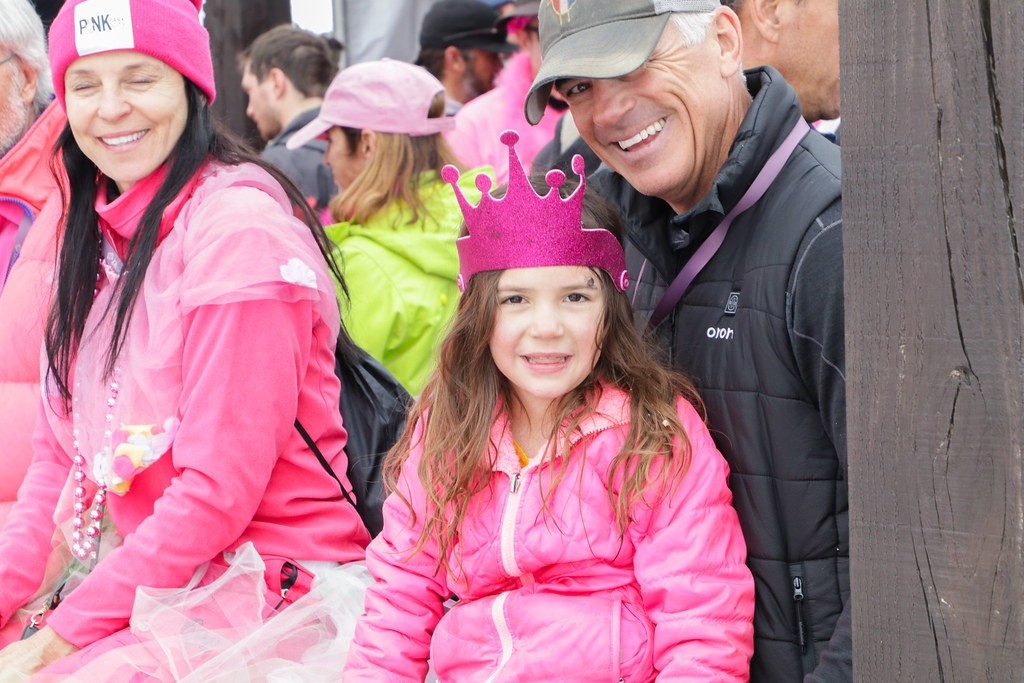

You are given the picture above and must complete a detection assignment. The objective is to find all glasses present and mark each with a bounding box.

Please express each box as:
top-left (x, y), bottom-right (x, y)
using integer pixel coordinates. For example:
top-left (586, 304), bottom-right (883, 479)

top-left (442, 26), bottom-right (510, 42)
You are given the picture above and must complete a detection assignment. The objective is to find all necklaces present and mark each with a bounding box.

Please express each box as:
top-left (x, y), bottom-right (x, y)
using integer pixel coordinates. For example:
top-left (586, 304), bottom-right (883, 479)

top-left (72, 238), bottom-right (126, 560)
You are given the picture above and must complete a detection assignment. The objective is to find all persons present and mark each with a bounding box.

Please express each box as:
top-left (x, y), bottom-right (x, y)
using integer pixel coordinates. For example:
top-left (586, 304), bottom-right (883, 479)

top-left (287, 57), bottom-right (499, 400)
top-left (342, 130), bottom-right (755, 682)
top-left (413, 0), bottom-right (852, 683)
top-left (0, 0), bottom-right (71, 526)
top-left (0, 0), bottom-right (371, 683)
top-left (241, 24), bottom-right (342, 228)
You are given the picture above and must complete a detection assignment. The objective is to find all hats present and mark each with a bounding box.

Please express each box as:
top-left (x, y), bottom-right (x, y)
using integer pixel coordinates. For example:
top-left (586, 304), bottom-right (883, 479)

top-left (287, 57), bottom-right (454, 150)
top-left (493, 0), bottom-right (538, 26)
top-left (48, 0), bottom-right (216, 114)
top-left (524, 0), bottom-right (721, 125)
top-left (420, 0), bottom-right (519, 56)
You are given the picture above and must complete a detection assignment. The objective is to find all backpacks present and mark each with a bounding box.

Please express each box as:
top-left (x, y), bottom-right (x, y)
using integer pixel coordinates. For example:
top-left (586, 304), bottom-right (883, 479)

top-left (295, 342), bottom-right (418, 538)
